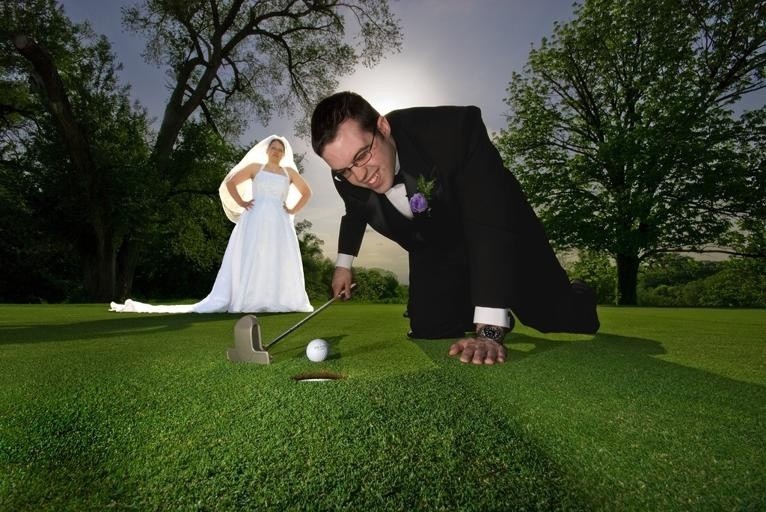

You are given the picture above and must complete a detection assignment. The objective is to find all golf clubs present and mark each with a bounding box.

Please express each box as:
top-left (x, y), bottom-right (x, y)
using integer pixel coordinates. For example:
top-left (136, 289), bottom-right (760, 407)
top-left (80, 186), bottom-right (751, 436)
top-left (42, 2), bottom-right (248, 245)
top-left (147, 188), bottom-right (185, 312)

top-left (225, 283), bottom-right (356, 362)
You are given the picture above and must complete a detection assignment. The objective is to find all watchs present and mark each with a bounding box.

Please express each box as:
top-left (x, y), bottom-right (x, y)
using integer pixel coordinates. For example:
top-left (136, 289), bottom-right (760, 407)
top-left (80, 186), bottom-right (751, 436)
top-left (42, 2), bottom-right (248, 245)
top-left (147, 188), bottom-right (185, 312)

top-left (472, 324), bottom-right (505, 344)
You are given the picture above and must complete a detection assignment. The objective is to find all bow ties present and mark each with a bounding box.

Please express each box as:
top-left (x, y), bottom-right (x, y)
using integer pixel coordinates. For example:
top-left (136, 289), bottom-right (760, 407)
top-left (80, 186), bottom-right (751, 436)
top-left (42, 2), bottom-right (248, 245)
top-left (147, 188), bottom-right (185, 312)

top-left (391, 174), bottom-right (404, 187)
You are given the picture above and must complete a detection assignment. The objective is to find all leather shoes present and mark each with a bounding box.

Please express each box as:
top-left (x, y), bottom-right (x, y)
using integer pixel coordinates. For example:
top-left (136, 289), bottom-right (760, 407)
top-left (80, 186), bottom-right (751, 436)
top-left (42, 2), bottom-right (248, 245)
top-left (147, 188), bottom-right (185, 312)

top-left (505, 311), bottom-right (515, 333)
top-left (570, 278), bottom-right (600, 333)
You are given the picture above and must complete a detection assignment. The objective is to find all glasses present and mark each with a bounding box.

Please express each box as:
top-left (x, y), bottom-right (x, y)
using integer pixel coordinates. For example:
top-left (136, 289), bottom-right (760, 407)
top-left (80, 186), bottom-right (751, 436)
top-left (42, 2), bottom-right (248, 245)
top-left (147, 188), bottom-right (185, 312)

top-left (332, 132), bottom-right (376, 183)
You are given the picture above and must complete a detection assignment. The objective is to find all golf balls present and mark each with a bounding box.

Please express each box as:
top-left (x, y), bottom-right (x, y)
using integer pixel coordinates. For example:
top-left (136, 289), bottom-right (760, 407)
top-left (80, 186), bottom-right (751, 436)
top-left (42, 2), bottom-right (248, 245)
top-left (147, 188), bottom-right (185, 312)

top-left (306, 339), bottom-right (329, 363)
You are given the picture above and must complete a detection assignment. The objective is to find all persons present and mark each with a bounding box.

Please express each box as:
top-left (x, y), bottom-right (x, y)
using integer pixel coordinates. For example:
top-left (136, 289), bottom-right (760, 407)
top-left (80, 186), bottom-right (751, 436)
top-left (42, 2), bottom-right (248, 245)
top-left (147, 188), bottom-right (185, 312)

top-left (310, 91), bottom-right (600, 367)
top-left (225, 139), bottom-right (312, 314)
